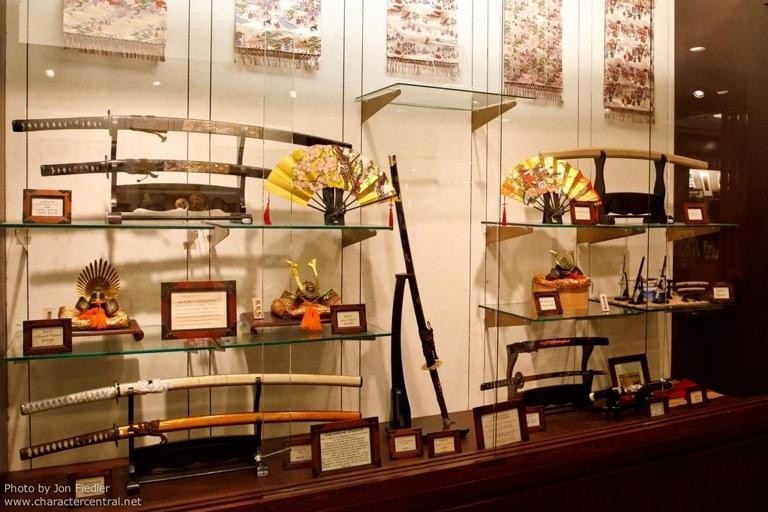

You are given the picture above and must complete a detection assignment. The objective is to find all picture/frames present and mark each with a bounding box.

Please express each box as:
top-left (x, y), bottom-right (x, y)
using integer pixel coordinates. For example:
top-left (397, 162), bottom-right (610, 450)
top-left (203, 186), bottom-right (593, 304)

top-left (570, 201), bottom-right (599, 224)
top-left (330, 304), bottom-right (367, 335)
top-left (160, 280), bottom-right (238, 340)
top-left (532, 291), bottom-right (563, 316)
top-left (22, 319), bottom-right (72, 356)
top-left (65, 468), bottom-right (114, 507)
top-left (608, 353), bottom-right (708, 419)
top-left (683, 202), bottom-right (709, 224)
top-left (709, 281), bottom-right (734, 302)
top-left (22, 189), bottom-right (72, 224)
top-left (284, 400), bottom-right (546, 479)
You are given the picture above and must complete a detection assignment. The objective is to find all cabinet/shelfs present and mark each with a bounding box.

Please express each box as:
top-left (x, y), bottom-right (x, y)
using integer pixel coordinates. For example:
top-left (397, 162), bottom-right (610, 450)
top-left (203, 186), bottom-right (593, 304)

top-left (0, 220), bottom-right (394, 361)
top-left (0, 380), bottom-right (768, 512)
top-left (481, 220), bottom-right (738, 322)
